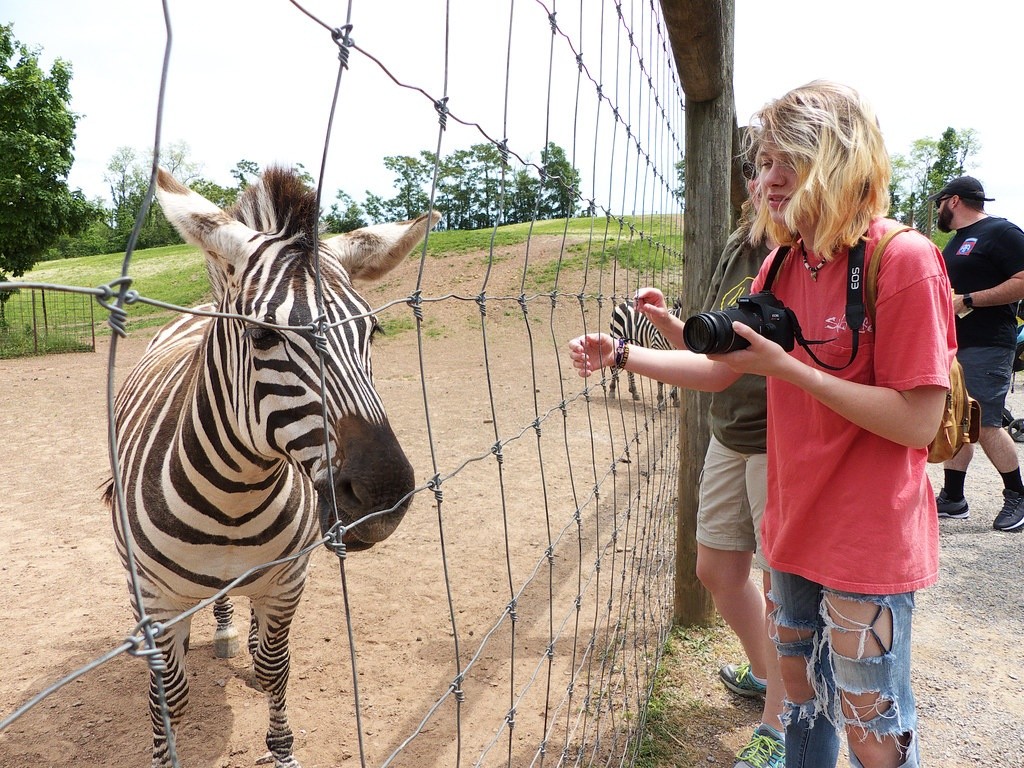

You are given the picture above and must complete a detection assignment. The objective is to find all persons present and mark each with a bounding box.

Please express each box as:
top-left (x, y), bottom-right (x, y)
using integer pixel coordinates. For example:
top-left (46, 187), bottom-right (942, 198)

top-left (568, 79), bottom-right (957, 768)
top-left (635, 126), bottom-right (791, 768)
top-left (925, 176), bottom-right (1024, 532)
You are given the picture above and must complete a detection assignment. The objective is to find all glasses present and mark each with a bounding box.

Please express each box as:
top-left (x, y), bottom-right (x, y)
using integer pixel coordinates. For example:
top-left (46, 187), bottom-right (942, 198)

top-left (935, 195), bottom-right (962, 208)
top-left (742, 161), bottom-right (756, 180)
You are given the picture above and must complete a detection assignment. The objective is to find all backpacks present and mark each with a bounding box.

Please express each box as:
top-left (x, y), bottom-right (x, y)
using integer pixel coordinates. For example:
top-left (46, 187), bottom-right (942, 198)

top-left (867, 224), bottom-right (982, 463)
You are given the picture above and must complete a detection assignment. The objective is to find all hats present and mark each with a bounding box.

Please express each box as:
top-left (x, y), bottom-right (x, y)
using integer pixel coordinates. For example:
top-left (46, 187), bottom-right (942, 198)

top-left (929, 175), bottom-right (995, 202)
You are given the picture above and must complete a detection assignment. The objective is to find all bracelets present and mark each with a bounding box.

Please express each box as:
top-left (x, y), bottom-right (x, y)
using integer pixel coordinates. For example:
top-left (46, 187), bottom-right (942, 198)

top-left (611, 337), bottom-right (642, 369)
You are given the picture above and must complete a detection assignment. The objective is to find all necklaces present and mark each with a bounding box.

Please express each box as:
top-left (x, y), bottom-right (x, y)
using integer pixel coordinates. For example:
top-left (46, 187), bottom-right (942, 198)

top-left (800, 238), bottom-right (827, 282)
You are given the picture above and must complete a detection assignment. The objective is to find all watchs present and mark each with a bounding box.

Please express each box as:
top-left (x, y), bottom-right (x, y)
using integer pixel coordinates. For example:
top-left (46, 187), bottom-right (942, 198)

top-left (963, 293), bottom-right (973, 309)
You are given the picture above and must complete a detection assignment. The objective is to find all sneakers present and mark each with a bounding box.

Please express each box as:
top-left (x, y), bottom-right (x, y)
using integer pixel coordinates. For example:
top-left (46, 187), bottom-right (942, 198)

top-left (732, 722), bottom-right (785, 768)
top-left (935, 488), bottom-right (969, 519)
top-left (720, 663), bottom-right (767, 697)
top-left (993, 489), bottom-right (1024, 531)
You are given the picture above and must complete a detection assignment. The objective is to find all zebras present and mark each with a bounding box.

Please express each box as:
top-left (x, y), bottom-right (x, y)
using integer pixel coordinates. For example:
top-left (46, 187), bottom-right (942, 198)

top-left (610, 298), bottom-right (683, 410)
top-left (99, 159), bottom-right (459, 768)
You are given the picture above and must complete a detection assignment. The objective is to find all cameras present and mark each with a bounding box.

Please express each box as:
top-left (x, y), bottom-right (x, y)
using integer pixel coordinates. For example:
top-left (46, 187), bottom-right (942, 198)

top-left (683, 291), bottom-right (795, 353)
